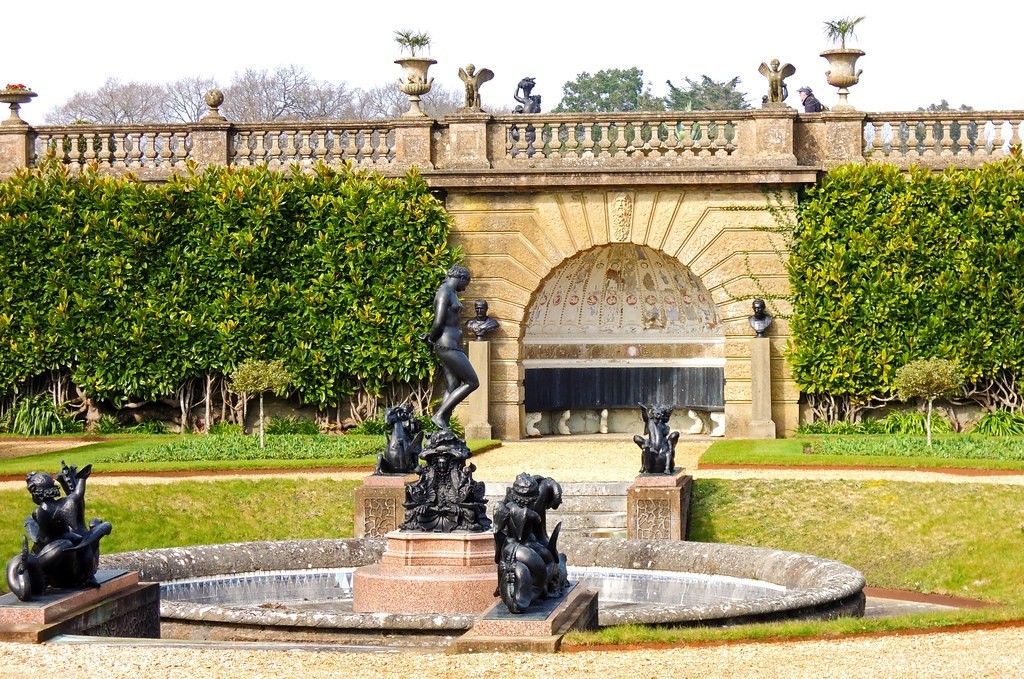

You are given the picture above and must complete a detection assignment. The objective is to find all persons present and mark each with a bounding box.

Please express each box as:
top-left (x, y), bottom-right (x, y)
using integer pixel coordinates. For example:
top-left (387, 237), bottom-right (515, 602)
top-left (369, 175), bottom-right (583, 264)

top-left (493, 472), bottom-right (555, 601)
top-left (748, 299), bottom-right (772, 333)
top-left (433, 456), bottom-right (458, 506)
top-left (796, 86), bottom-right (821, 113)
top-left (25, 470), bottom-right (91, 554)
top-left (464, 64), bottom-right (479, 107)
top-left (513, 88), bottom-right (541, 113)
top-left (768, 59), bottom-right (783, 103)
top-left (639, 405), bottom-right (671, 475)
top-left (419, 266), bottom-right (480, 430)
top-left (465, 299), bottom-right (499, 336)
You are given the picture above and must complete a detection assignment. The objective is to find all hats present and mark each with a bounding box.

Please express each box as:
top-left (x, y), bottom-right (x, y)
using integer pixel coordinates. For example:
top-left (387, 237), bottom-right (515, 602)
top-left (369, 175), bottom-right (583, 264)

top-left (796, 86), bottom-right (812, 92)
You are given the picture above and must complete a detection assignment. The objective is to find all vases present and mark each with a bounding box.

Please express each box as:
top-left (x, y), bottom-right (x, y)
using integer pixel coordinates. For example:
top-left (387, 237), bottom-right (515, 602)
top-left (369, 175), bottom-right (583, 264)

top-left (0, 90), bottom-right (38, 126)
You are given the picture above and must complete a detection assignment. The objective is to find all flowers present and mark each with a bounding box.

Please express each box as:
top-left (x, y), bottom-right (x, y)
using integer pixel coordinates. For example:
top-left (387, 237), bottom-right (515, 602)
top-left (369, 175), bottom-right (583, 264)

top-left (3, 83), bottom-right (27, 89)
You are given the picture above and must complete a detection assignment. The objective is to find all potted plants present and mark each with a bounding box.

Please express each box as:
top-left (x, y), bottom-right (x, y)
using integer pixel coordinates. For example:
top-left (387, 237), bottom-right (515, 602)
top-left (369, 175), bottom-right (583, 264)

top-left (390, 30), bottom-right (436, 116)
top-left (822, 18), bottom-right (869, 112)
